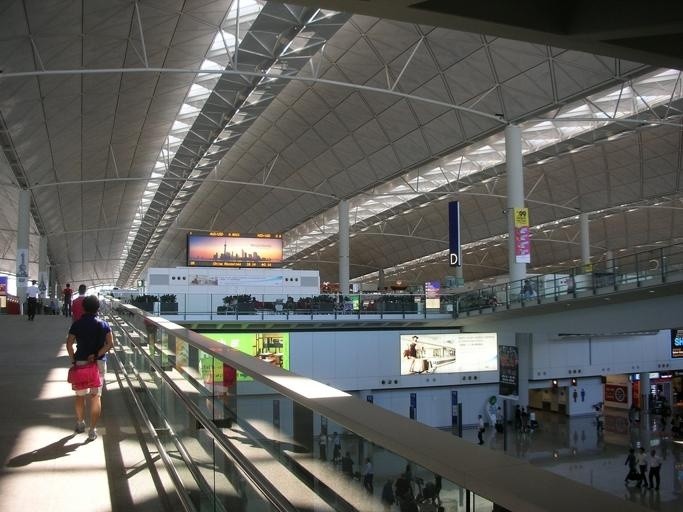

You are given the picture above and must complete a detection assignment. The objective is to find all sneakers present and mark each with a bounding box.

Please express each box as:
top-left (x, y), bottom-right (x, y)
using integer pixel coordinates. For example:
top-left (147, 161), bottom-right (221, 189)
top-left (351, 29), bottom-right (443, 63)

top-left (87, 427), bottom-right (97, 441)
top-left (73, 420), bottom-right (86, 433)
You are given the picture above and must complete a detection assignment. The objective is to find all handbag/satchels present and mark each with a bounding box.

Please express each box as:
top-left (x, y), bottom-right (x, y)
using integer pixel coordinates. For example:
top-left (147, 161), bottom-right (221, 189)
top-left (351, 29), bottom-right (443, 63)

top-left (629, 471), bottom-right (640, 480)
top-left (67, 360), bottom-right (102, 390)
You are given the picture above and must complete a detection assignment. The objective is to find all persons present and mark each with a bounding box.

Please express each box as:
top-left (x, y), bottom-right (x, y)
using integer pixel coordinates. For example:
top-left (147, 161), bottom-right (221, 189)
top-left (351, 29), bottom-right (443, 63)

top-left (486, 295), bottom-right (497, 305)
top-left (520, 408), bottom-right (528, 433)
top-left (63, 284), bottom-right (73, 317)
top-left (69, 284), bottom-right (87, 320)
top-left (315, 427), bottom-right (447, 511)
top-left (561, 274), bottom-right (576, 298)
top-left (647, 449), bottom-right (663, 490)
top-left (624, 448), bottom-right (639, 482)
top-left (519, 280), bottom-right (533, 296)
top-left (496, 406), bottom-right (504, 432)
top-left (635, 446), bottom-right (648, 488)
top-left (409, 336), bottom-right (418, 357)
top-left (25, 280), bottom-right (41, 321)
top-left (476, 414), bottom-right (485, 445)
top-left (66, 294), bottom-right (114, 440)
top-left (514, 404), bottom-right (522, 432)
